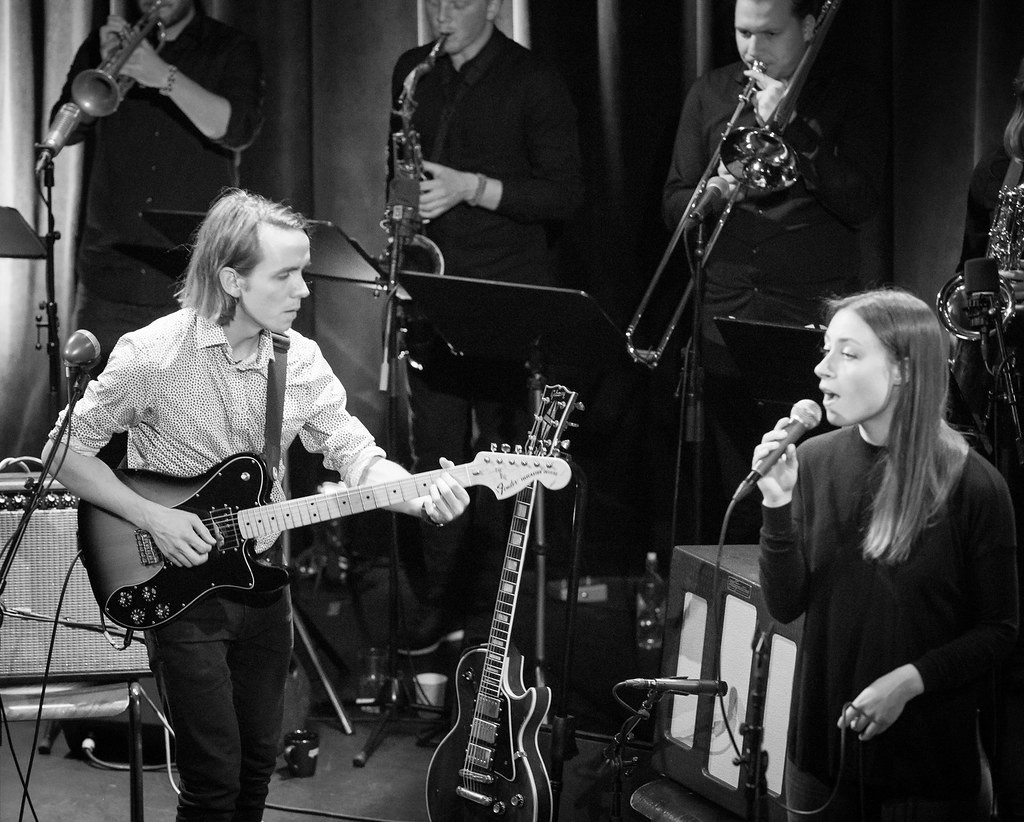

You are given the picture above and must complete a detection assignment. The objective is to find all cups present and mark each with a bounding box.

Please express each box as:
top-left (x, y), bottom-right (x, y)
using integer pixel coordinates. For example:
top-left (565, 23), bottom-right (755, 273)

top-left (284, 729), bottom-right (320, 776)
top-left (412, 672), bottom-right (448, 718)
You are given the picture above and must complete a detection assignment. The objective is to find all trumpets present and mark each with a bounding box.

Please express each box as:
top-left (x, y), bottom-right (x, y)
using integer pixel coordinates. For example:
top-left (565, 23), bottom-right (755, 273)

top-left (70, 1), bottom-right (168, 119)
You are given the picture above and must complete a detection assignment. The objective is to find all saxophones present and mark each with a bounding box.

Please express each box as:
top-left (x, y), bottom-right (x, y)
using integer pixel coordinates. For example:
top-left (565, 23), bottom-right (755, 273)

top-left (380, 31), bottom-right (447, 276)
top-left (937, 183), bottom-right (1024, 415)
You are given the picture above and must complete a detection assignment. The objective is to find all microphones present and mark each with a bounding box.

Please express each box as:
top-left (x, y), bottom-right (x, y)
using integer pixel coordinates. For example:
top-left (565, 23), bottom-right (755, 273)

top-left (624, 679), bottom-right (728, 697)
top-left (34, 102), bottom-right (83, 172)
top-left (63, 330), bottom-right (101, 365)
top-left (964, 259), bottom-right (1000, 361)
top-left (732, 399), bottom-right (822, 502)
top-left (386, 177), bottom-right (422, 248)
top-left (682, 177), bottom-right (729, 230)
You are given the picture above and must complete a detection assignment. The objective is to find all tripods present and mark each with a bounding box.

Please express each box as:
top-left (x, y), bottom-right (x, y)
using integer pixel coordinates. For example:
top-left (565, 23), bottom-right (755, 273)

top-left (323, 238), bottom-right (453, 767)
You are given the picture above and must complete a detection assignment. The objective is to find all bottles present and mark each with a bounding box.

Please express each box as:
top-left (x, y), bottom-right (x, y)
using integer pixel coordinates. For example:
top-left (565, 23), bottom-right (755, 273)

top-left (361, 646), bottom-right (384, 699)
top-left (636, 553), bottom-right (665, 649)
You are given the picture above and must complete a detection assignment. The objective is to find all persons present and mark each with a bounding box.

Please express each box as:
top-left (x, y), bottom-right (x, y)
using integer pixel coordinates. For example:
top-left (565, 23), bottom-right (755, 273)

top-left (386, 0), bottom-right (587, 653)
top-left (750, 290), bottom-right (1019, 821)
top-left (41, 191), bottom-right (472, 821)
top-left (48, 0), bottom-right (268, 470)
top-left (946, 56), bottom-right (1023, 497)
top-left (664, 0), bottom-right (887, 542)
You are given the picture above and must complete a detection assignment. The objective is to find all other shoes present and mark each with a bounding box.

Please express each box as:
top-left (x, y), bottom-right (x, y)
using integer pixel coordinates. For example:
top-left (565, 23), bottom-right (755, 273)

top-left (397, 610), bottom-right (464, 655)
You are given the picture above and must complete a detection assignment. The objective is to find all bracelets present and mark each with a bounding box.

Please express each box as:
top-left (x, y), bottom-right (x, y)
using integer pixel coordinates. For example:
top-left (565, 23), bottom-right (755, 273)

top-left (468, 175), bottom-right (486, 206)
top-left (160, 65), bottom-right (177, 95)
top-left (421, 505), bottom-right (443, 527)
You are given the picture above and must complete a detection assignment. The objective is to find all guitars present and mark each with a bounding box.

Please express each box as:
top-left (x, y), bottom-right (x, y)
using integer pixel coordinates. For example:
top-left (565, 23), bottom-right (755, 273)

top-left (79, 437), bottom-right (576, 630)
top-left (420, 380), bottom-right (590, 820)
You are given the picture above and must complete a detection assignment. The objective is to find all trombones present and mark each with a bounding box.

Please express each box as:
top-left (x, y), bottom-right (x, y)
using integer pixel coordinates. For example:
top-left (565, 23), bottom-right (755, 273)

top-left (625, 1), bottom-right (838, 366)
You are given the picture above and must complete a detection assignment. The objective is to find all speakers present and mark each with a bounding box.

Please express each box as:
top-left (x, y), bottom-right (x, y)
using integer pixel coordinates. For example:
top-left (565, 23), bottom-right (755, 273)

top-left (653, 542), bottom-right (805, 822)
top-left (0, 469), bottom-right (154, 683)
